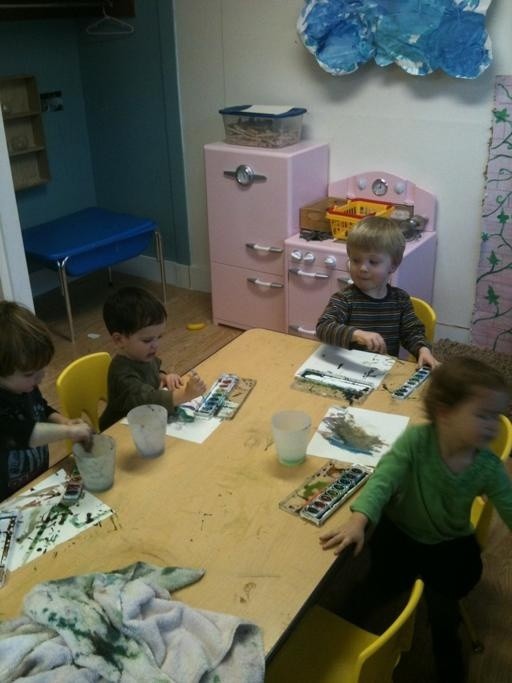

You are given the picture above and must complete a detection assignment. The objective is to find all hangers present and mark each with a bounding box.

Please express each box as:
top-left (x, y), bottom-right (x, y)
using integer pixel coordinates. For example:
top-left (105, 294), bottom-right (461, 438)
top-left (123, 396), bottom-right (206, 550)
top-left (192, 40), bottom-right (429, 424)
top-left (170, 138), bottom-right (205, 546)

top-left (85, 3), bottom-right (134, 36)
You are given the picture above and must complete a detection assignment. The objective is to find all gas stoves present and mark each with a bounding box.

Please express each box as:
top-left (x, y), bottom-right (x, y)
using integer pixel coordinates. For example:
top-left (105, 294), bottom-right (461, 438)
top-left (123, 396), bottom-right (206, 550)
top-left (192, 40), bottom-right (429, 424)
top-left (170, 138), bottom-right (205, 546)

top-left (284, 226), bottom-right (436, 260)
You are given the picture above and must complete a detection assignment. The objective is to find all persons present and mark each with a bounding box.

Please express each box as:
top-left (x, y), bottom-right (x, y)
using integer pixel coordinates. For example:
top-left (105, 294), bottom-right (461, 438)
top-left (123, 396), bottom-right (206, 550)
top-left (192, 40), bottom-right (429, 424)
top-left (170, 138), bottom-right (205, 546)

top-left (315, 217), bottom-right (440, 371)
top-left (1, 300), bottom-right (93, 503)
top-left (318, 357), bottom-right (512, 603)
top-left (96, 286), bottom-right (208, 432)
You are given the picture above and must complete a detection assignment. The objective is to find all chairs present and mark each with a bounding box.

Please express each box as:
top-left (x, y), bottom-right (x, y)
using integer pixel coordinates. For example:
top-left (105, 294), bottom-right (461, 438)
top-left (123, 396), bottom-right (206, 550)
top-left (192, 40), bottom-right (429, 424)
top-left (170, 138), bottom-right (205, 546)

top-left (399, 296), bottom-right (437, 361)
top-left (56, 352), bottom-right (112, 455)
top-left (266, 580), bottom-right (437, 681)
top-left (464, 411), bottom-right (510, 540)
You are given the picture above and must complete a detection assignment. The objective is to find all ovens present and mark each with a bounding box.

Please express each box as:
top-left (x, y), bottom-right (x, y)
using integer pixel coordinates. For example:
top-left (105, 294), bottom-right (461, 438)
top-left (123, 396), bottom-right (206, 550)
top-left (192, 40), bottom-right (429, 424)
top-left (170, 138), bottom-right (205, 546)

top-left (283, 242), bottom-right (398, 343)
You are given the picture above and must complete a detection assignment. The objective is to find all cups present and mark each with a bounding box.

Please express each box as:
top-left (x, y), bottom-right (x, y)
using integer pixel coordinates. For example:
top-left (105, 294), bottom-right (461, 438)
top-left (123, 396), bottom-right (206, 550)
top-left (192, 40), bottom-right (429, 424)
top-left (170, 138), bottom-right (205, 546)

top-left (71, 433), bottom-right (118, 495)
top-left (269, 408), bottom-right (313, 467)
top-left (127, 403), bottom-right (168, 460)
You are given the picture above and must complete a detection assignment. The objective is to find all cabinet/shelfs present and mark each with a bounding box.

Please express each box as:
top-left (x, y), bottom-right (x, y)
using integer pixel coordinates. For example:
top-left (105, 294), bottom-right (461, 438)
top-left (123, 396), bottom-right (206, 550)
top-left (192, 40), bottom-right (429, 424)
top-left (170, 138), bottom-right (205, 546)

top-left (20, 208), bottom-right (167, 345)
top-left (203, 136), bottom-right (329, 333)
top-left (287, 232), bottom-right (436, 337)
top-left (3, 79), bottom-right (49, 192)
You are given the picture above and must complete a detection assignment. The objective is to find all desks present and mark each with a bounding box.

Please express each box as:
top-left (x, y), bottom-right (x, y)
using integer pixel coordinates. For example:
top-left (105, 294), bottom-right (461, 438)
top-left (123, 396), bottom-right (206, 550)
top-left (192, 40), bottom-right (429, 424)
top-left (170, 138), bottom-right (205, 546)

top-left (0, 327), bottom-right (457, 682)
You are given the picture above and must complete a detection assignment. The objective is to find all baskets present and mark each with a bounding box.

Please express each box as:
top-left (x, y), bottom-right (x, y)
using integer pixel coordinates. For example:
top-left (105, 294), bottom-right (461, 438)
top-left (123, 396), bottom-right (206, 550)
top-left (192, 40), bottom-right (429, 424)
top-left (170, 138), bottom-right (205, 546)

top-left (325, 198), bottom-right (395, 240)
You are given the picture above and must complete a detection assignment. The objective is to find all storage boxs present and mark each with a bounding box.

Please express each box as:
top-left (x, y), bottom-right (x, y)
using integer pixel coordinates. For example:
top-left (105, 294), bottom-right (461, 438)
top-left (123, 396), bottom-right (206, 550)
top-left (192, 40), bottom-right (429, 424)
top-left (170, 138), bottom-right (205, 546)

top-left (215, 103), bottom-right (307, 145)
